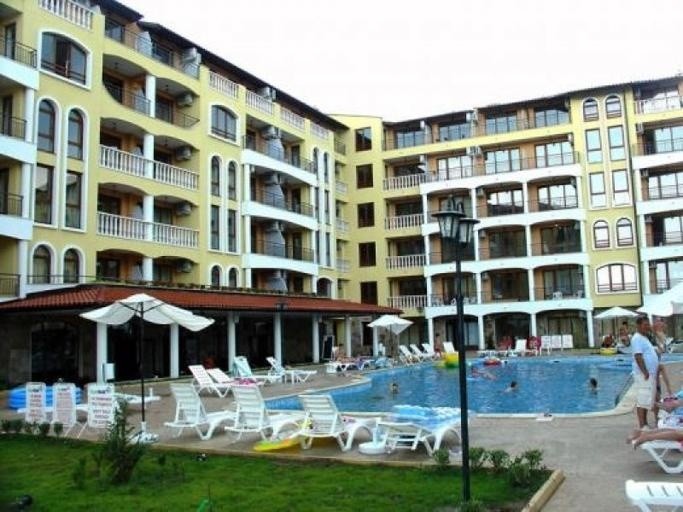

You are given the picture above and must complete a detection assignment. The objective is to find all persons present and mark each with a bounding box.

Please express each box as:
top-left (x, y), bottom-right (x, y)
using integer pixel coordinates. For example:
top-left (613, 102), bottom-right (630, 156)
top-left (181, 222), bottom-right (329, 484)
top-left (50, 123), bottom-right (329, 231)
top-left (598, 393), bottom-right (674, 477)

top-left (654, 397), bottom-right (682, 410)
top-left (377, 328), bottom-right (402, 361)
top-left (617, 327), bottom-right (628, 349)
top-left (621, 321), bottom-right (629, 339)
top-left (653, 320), bottom-right (667, 344)
top-left (470, 365), bottom-right (498, 381)
top-left (333, 343), bottom-right (361, 363)
top-left (387, 382), bottom-right (401, 394)
top-left (602, 332), bottom-right (616, 348)
top-left (654, 364), bottom-right (672, 424)
top-left (434, 333), bottom-right (442, 362)
top-left (631, 316), bottom-right (659, 429)
top-left (588, 376), bottom-right (601, 398)
top-left (495, 334), bottom-right (540, 351)
top-left (503, 379), bottom-right (519, 395)
top-left (624, 425), bottom-right (682, 451)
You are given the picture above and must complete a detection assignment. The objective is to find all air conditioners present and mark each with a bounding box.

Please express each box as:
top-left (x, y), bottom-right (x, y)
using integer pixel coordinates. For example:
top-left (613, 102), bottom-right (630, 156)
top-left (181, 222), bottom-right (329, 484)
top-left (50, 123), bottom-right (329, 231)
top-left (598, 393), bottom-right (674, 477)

top-left (172, 59), bottom-right (194, 275)
top-left (256, 86), bottom-right (287, 283)
top-left (561, 97), bottom-right (582, 294)
top-left (631, 88), bottom-right (656, 271)
top-left (462, 110), bottom-right (490, 282)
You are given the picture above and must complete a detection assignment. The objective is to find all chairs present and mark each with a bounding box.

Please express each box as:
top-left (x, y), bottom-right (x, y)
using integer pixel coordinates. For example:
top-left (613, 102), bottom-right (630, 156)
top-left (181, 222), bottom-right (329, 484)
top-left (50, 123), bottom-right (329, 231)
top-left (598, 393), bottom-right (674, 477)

top-left (376, 416), bottom-right (456, 458)
top-left (295, 392), bottom-right (374, 453)
top-left (472, 333), bottom-right (575, 358)
top-left (162, 381), bottom-right (239, 442)
top-left (222, 383), bottom-right (305, 444)
top-left (625, 391), bottom-right (682, 512)
top-left (18, 381), bottom-right (123, 441)
top-left (183, 341), bottom-right (458, 394)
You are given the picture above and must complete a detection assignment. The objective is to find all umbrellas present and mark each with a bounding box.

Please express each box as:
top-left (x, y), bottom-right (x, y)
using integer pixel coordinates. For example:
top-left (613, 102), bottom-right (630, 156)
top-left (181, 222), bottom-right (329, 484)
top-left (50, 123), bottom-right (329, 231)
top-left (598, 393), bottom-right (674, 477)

top-left (77, 292), bottom-right (213, 435)
top-left (636, 279), bottom-right (682, 318)
top-left (593, 305), bottom-right (637, 346)
top-left (366, 314), bottom-right (415, 358)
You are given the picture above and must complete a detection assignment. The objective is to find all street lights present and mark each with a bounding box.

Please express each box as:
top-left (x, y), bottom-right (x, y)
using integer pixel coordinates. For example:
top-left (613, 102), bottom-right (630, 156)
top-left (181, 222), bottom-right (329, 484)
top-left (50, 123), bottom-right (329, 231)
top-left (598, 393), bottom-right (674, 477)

top-left (432, 195), bottom-right (484, 496)
